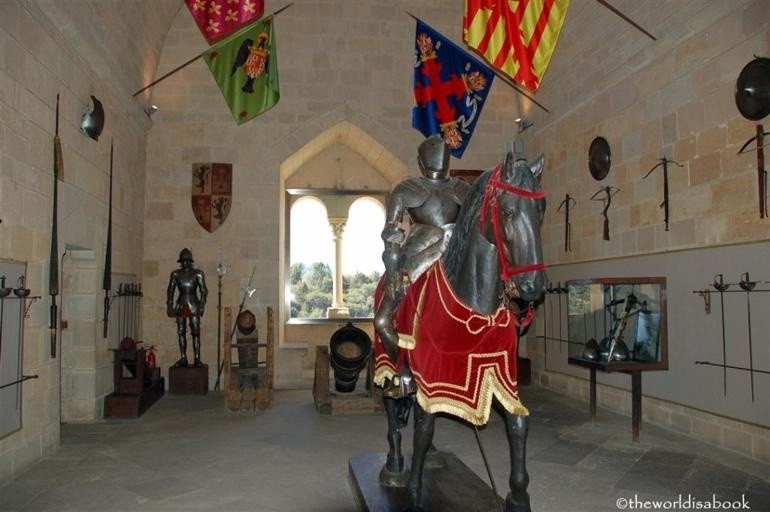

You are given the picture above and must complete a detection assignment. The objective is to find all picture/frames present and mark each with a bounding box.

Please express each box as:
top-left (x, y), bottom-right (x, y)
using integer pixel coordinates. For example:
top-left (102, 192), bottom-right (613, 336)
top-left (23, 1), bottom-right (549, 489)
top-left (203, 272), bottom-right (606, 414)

top-left (569, 357), bottom-right (663, 444)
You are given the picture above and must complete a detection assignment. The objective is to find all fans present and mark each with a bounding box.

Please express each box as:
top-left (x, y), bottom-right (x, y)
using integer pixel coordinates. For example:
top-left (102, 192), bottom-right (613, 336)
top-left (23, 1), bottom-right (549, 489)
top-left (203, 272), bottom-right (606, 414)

top-left (0, 258), bottom-right (27, 441)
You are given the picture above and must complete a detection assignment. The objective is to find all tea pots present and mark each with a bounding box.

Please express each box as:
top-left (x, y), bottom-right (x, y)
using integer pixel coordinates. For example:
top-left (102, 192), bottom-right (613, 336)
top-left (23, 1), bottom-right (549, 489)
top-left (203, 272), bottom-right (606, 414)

top-left (564, 275), bottom-right (668, 372)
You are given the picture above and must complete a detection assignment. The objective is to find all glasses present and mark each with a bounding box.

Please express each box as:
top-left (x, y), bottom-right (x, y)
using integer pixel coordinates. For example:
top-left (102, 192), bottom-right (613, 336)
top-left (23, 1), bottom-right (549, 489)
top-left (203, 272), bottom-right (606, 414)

top-left (514, 116), bottom-right (532, 133)
top-left (145, 104), bottom-right (159, 117)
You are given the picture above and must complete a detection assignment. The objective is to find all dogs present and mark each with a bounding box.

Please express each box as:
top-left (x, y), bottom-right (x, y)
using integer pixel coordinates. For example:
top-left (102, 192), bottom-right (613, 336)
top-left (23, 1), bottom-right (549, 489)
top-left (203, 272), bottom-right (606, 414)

top-left (377, 150), bottom-right (548, 512)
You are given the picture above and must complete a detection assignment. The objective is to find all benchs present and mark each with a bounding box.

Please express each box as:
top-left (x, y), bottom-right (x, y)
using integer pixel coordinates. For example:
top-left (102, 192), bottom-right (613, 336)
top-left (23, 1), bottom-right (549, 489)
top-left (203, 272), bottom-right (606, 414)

top-left (186, 0), bottom-right (263, 46)
top-left (462, 2), bottom-right (568, 95)
top-left (200, 14), bottom-right (280, 128)
top-left (411, 19), bottom-right (495, 160)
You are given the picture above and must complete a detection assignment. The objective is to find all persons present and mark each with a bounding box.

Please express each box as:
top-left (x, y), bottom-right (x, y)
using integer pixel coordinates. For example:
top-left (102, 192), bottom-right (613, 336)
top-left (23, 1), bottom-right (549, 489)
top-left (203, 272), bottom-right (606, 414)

top-left (372, 135), bottom-right (474, 398)
top-left (165, 246), bottom-right (209, 368)
